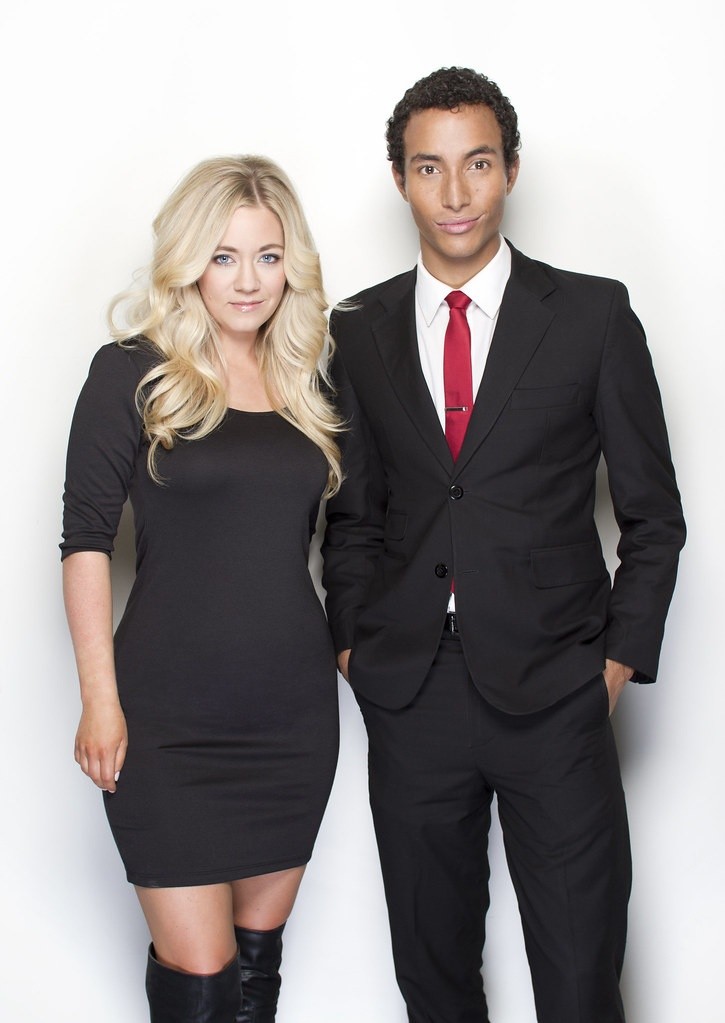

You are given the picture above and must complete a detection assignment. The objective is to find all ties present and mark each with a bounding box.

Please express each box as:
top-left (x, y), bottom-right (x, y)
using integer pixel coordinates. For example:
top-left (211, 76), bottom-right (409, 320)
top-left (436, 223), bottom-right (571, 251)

top-left (443, 290), bottom-right (474, 594)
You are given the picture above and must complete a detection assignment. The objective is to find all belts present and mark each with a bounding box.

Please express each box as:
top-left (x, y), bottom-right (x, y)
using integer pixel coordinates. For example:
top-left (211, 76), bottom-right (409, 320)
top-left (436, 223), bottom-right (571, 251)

top-left (444, 613), bottom-right (460, 637)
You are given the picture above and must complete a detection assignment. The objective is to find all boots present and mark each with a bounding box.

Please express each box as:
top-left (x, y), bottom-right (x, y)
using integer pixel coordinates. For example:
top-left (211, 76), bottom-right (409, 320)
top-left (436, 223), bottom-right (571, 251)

top-left (146, 941), bottom-right (243, 1023)
top-left (234, 921), bottom-right (287, 1023)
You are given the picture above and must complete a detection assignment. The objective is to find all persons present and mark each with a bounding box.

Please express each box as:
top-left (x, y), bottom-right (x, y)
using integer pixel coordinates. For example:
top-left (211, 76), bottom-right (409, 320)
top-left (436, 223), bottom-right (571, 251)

top-left (318, 68), bottom-right (688, 1023)
top-left (57, 154), bottom-right (356, 1023)
